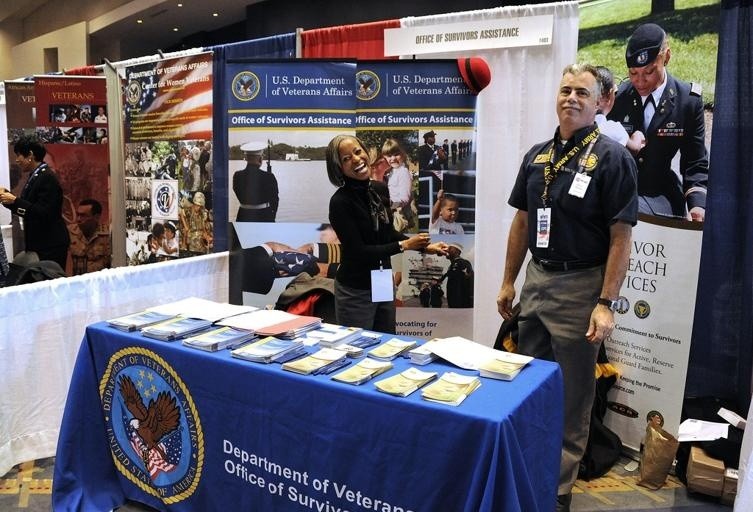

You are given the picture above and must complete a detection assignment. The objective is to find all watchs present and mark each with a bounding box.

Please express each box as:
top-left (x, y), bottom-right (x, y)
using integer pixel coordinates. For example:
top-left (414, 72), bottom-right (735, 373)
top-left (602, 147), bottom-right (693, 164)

top-left (596, 298), bottom-right (620, 312)
top-left (398, 240), bottom-right (404, 252)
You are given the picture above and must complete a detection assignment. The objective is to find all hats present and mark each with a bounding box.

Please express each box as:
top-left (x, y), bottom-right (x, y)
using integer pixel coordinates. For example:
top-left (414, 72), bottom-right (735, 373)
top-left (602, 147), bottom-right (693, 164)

top-left (625, 23), bottom-right (665, 68)
top-left (423, 130), bottom-right (437, 137)
top-left (239, 140), bottom-right (269, 156)
top-left (457, 57), bottom-right (491, 96)
top-left (449, 241), bottom-right (464, 253)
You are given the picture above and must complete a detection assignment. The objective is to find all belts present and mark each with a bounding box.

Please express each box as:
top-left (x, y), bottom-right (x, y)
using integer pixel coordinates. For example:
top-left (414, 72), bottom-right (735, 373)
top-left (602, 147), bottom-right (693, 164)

top-left (532, 255), bottom-right (605, 272)
top-left (239, 202), bottom-right (270, 210)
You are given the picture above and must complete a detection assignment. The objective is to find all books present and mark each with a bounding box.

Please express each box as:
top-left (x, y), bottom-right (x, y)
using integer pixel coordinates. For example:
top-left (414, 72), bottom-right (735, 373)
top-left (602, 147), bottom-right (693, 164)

top-left (232, 337), bottom-right (305, 362)
top-left (182, 328), bottom-right (254, 353)
top-left (142, 319), bottom-right (211, 343)
top-left (305, 323), bottom-right (361, 346)
top-left (108, 313), bottom-right (172, 332)
top-left (375, 365), bottom-right (436, 396)
top-left (480, 348), bottom-right (535, 383)
top-left (435, 339), bottom-right (483, 367)
top-left (368, 337), bottom-right (417, 362)
top-left (150, 299), bottom-right (257, 324)
top-left (332, 359), bottom-right (391, 385)
top-left (348, 336), bottom-right (381, 349)
top-left (405, 337), bottom-right (444, 366)
top-left (283, 348), bottom-right (346, 377)
top-left (213, 309), bottom-right (321, 339)
top-left (423, 372), bottom-right (482, 407)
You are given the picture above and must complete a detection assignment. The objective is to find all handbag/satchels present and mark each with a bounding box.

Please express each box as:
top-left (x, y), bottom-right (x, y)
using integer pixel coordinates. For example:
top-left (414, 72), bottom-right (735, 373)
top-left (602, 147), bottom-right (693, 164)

top-left (634, 415), bottom-right (681, 492)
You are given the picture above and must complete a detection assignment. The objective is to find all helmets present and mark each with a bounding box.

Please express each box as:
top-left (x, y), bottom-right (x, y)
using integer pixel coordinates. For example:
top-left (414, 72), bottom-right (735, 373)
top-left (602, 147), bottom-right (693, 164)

top-left (193, 192), bottom-right (206, 207)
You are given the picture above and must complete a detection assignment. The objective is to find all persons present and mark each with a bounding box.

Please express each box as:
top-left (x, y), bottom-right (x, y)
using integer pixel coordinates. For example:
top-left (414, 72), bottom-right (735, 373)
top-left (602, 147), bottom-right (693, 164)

top-left (497, 63), bottom-right (638, 512)
top-left (440, 241), bottom-right (474, 309)
top-left (0, 137), bottom-right (70, 271)
top-left (585, 65), bottom-right (644, 149)
top-left (382, 138), bottom-right (415, 227)
top-left (8, 104), bottom-right (109, 145)
top-left (325, 133), bottom-right (451, 335)
top-left (233, 142), bottom-right (279, 222)
top-left (611, 24), bottom-right (710, 222)
top-left (421, 131), bottom-right (475, 170)
top-left (67, 199), bottom-right (111, 277)
top-left (429, 188), bottom-right (466, 234)
top-left (125, 140), bottom-right (213, 267)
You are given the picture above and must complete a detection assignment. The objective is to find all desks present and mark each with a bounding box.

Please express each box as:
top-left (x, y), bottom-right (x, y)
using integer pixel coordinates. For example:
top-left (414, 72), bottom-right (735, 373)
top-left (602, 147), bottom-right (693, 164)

top-left (52, 299), bottom-right (563, 512)
